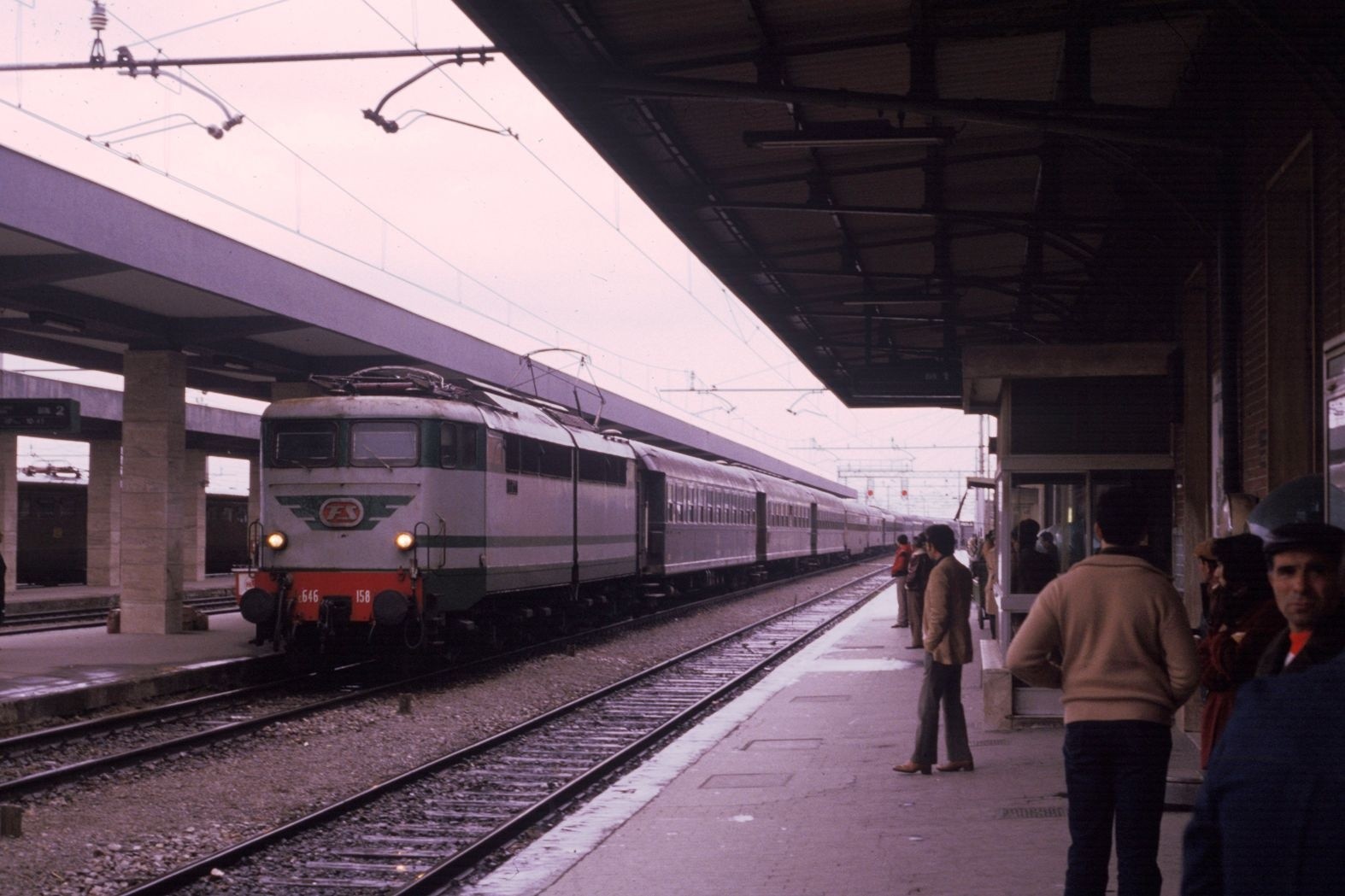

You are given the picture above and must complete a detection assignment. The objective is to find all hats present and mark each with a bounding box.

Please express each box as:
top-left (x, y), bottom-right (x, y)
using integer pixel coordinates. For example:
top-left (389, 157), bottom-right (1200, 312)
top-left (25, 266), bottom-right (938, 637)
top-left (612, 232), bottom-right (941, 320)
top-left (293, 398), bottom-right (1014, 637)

top-left (1187, 537), bottom-right (1224, 561)
top-left (1260, 522), bottom-right (1345, 550)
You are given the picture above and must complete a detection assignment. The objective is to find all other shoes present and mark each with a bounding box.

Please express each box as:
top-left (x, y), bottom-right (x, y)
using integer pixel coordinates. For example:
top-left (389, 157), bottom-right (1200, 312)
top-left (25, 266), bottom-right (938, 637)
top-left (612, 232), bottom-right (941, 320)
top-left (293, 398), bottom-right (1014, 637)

top-left (905, 645), bottom-right (924, 649)
top-left (892, 624), bottom-right (909, 628)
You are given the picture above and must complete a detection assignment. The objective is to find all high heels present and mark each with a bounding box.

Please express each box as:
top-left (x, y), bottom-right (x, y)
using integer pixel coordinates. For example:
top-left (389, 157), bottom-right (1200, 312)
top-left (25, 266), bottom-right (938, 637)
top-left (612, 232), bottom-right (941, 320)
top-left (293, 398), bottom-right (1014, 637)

top-left (936, 758), bottom-right (974, 771)
top-left (892, 760), bottom-right (932, 775)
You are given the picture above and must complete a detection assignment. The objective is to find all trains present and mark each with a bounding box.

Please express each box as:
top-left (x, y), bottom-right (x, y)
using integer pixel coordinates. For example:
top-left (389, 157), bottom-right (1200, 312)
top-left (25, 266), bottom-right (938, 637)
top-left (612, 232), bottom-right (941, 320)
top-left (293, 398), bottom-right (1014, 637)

top-left (236, 360), bottom-right (926, 680)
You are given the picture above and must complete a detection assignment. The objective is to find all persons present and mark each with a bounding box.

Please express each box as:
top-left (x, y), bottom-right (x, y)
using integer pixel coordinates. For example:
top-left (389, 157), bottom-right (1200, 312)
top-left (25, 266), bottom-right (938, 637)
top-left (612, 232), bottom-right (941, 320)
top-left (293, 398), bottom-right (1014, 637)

top-left (893, 524), bottom-right (973, 774)
top-left (968, 519), bottom-right (1059, 639)
top-left (1180, 523), bottom-right (1345, 896)
top-left (1007, 488), bottom-right (1201, 896)
top-left (890, 534), bottom-right (932, 648)
